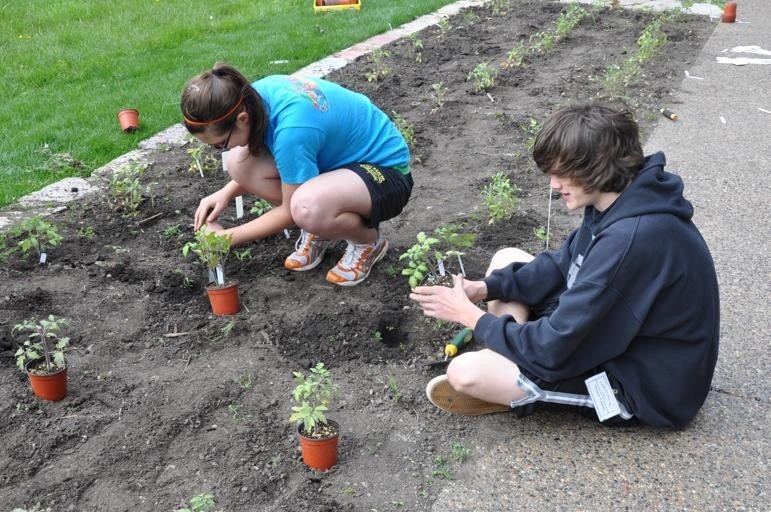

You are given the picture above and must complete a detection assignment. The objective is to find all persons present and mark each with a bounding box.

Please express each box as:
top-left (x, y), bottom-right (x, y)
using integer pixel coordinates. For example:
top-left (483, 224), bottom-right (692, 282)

top-left (411, 101), bottom-right (721, 428)
top-left (180, 61), bottom-right (413, 285)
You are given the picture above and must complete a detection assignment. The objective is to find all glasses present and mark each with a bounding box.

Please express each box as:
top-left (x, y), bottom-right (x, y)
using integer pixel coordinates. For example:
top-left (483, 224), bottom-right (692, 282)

top-left (213, 124), bottom-right (234, 152)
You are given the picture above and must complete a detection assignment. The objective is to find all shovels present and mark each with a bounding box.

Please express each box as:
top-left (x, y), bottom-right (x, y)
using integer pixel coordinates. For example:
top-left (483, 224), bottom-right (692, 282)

top-left (423, 327), bottom-right (474, 366)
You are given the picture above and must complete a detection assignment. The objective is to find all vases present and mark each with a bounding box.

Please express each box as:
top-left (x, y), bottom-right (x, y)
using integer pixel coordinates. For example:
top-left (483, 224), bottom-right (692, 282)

top-left (316, 0), bottom-right (357, 6)
top-left (722, 2), bottom-right (736, 23)
top-left (117, 109), bottom-right (139, 133)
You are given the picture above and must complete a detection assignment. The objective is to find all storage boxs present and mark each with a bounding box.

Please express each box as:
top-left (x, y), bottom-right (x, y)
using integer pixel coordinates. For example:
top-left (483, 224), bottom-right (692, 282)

top-left (313, 0), bottom-right (361, 15)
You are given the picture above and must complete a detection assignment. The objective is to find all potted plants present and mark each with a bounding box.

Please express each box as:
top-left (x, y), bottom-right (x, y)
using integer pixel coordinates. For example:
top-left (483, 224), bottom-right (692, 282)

top-left (11, 314), bottom-right (71, 402)
top-left (398, 231), bottom-right (454, 295)
top-left (288, 363), bottom-right (339, 472)
top-left (183, 224), bottom-right (240, 316)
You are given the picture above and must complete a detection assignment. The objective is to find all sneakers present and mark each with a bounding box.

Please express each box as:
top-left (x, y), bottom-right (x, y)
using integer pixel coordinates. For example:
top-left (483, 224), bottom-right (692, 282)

top-left (284, 228), bottom-right (335, 273)
top-left (325, 231), bottom-right (389, 287)
top-left (425, 368), bottom-right (513, 417)
